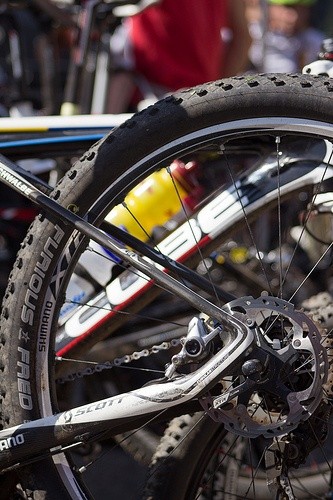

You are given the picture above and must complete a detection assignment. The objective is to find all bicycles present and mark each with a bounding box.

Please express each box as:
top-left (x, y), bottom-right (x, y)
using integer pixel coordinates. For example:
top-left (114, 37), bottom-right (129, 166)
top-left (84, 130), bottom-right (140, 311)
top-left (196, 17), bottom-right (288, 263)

top-left (0, 1), bottom-right (332, 500)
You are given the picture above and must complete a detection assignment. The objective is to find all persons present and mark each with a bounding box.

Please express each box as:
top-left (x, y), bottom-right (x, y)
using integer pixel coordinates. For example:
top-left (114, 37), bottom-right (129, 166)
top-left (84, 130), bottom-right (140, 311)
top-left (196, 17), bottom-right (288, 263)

top-left (107, 0), bottom-right (254, 115)
